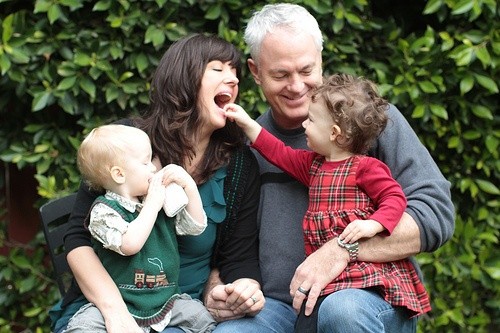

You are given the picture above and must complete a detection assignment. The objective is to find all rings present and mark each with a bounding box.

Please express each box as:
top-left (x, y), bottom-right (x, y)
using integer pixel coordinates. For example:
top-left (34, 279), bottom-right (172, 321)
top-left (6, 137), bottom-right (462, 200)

top-left (248, 296), bottom-right (257, 303)
top-left (297, 284), bottom-right (308, 295)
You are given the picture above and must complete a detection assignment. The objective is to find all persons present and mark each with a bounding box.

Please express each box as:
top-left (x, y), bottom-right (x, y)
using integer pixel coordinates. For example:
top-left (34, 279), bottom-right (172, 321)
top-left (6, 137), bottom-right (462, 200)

top-left (59, 123), bottom-right (218, 333)
top-left (50, 31), bottom-right (264, 333)
top-left (223, 70), bottom-right (435, 333)
top-left (211, 3), bottom-right (457, 333)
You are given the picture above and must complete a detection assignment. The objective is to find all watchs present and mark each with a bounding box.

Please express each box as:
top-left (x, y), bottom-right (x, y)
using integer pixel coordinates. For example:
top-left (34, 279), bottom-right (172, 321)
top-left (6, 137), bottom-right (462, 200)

top-left (337, 234), bottom-right (358, 264)
